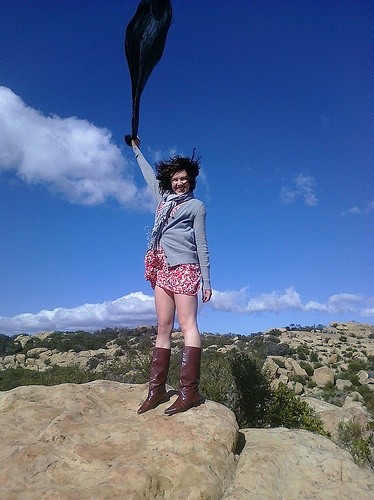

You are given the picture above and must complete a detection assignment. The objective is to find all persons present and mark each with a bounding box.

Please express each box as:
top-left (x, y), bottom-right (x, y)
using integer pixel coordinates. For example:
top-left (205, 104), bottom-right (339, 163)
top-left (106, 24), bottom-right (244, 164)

top-left (129, 136), bottom-right (213, 414)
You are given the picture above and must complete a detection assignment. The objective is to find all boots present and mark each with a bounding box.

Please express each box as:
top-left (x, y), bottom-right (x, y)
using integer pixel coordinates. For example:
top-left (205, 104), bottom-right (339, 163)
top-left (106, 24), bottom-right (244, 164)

top-left (137, 347), bottom-right (171, 414)
top-left (164, 346), bottom-right (204, 414)
